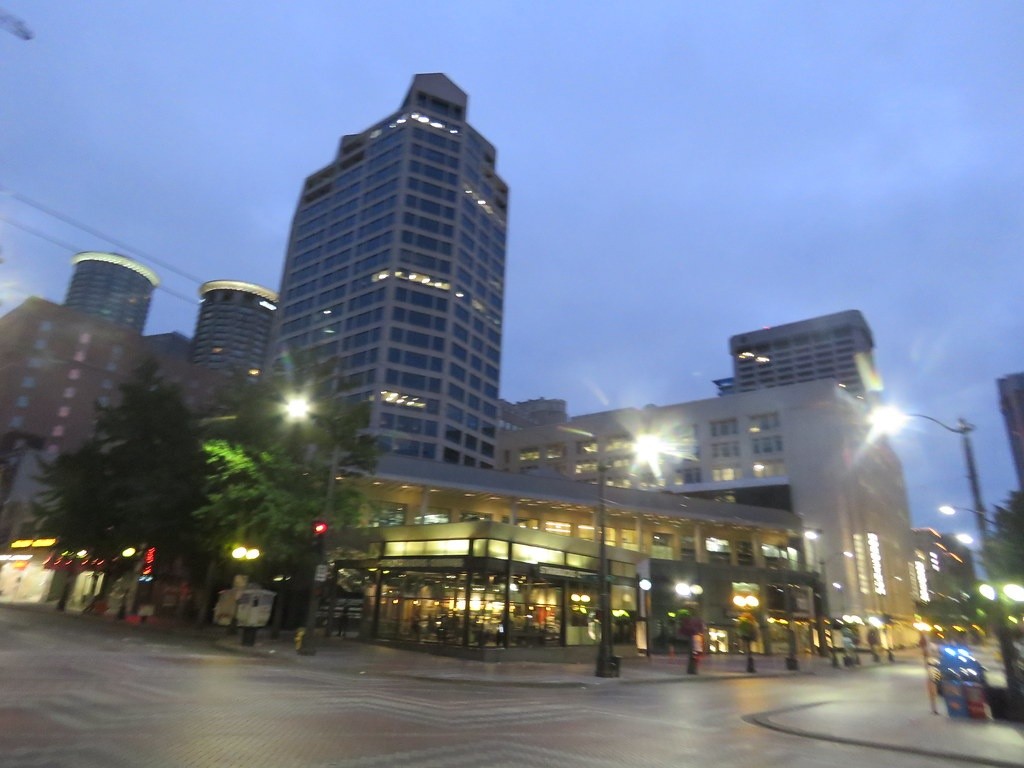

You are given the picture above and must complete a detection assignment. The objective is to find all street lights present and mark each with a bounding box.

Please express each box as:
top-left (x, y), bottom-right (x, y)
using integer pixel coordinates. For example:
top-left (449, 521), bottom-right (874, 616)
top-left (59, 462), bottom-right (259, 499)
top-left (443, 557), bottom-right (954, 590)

top-left (582, 433), bottom-right (665, 680)
top-left (864, 405), bottom-right (1023, 717)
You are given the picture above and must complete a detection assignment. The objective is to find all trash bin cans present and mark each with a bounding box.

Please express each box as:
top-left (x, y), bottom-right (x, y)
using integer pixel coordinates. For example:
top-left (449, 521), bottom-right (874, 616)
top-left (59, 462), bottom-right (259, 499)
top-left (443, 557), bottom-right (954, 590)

top-left (942, 679), bottom-right (968, 718)
top-left (961, 681), bottom-right (986, 720)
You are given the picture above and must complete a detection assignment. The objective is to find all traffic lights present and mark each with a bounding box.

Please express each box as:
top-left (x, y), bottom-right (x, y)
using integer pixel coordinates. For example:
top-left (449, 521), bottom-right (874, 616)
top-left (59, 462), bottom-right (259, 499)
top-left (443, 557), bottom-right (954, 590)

top-left (141, 546), bottom-right (155, 582)
top-left (312, 520), bottom-right (326, 536)
